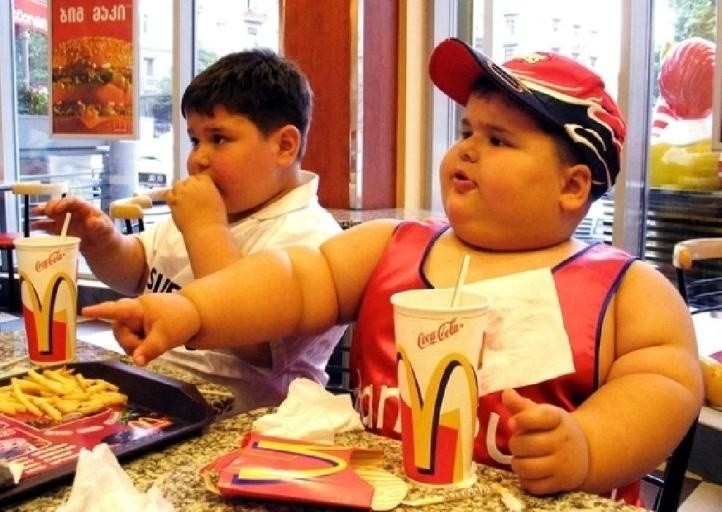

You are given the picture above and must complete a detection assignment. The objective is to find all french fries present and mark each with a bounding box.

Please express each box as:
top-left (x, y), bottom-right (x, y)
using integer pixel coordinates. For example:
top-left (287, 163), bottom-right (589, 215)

top-left (0, 367), bottom-right (127, 422)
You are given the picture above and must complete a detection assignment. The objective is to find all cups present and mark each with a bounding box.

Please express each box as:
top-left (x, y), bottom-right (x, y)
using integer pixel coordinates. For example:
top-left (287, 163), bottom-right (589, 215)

top-left (14, 237), bottom-right (81, 362)
top-left (390, 288), bottom-right (489, 485)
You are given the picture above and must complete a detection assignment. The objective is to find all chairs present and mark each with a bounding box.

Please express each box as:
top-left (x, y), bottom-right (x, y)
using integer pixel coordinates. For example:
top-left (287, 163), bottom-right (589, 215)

top-left (109, 195), bottom-right (153, 233)
top-left (651, 238), bottom-right (722, 512)
top-left (0, 181), bottom-right (68, 313)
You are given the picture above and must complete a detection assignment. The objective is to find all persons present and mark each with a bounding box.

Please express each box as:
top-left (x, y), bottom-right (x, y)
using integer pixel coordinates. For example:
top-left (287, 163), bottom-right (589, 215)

top-left (22, 48), bottom-right (351, 416)
top-left (75, 36), bottom-right (708, 508)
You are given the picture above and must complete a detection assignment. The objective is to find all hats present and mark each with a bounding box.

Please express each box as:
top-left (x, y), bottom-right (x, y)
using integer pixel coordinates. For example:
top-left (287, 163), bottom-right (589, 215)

top-left (428, 36), bottom-right (626, 197)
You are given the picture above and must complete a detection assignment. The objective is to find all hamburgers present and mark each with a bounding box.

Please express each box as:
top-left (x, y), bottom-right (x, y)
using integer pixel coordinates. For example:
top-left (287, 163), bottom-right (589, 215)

top-left (53, 37), bottom-right (133, 134)
